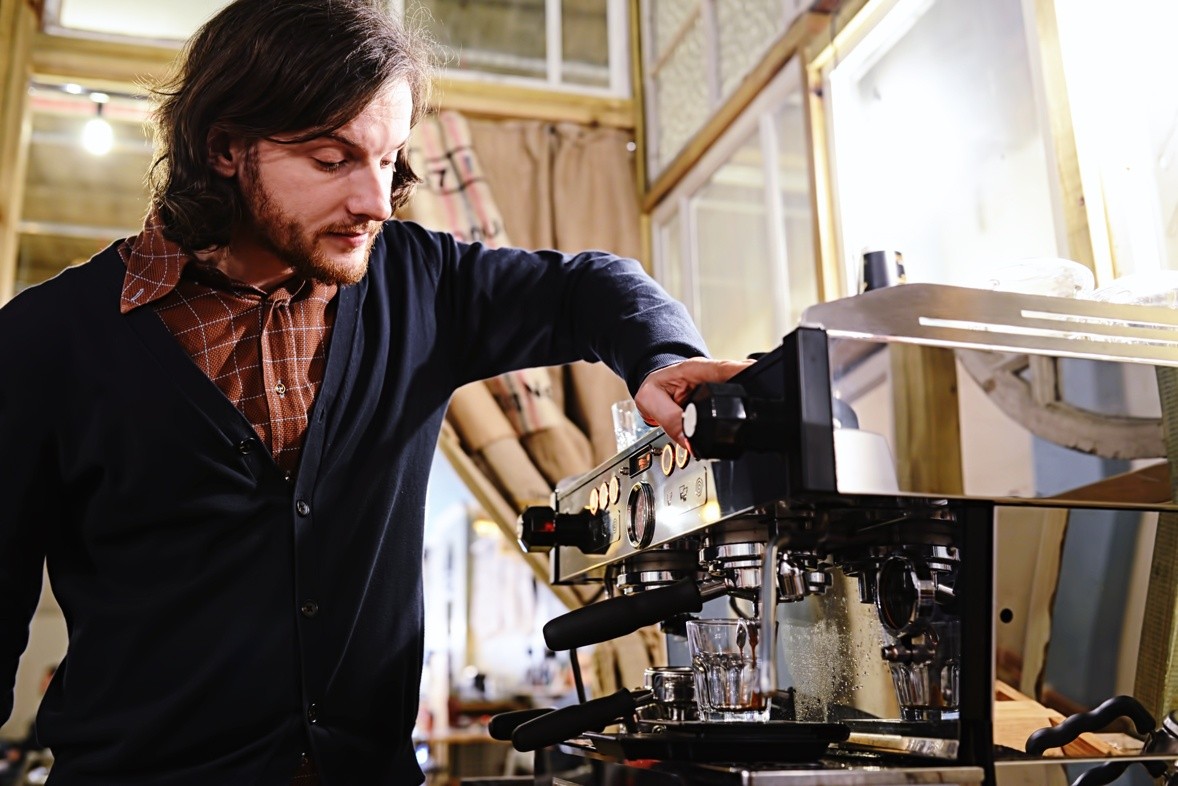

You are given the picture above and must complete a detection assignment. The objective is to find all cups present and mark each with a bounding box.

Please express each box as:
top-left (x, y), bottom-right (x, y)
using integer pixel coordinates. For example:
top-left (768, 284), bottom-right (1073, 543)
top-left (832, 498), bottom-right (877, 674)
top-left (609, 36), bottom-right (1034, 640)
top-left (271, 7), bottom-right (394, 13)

top-left (611, 397), bottom-right (651, 461)
top-left (685, 619), bottom-right (774, 722)
top-left (864, 251), bottom-right (906, 294)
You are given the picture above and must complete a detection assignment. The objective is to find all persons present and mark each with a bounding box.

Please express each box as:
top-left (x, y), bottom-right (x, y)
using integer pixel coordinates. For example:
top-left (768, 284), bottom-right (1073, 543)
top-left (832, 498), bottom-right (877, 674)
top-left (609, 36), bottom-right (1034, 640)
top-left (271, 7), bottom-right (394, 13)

top-left (0, 0), bottom-right (766, 786)
